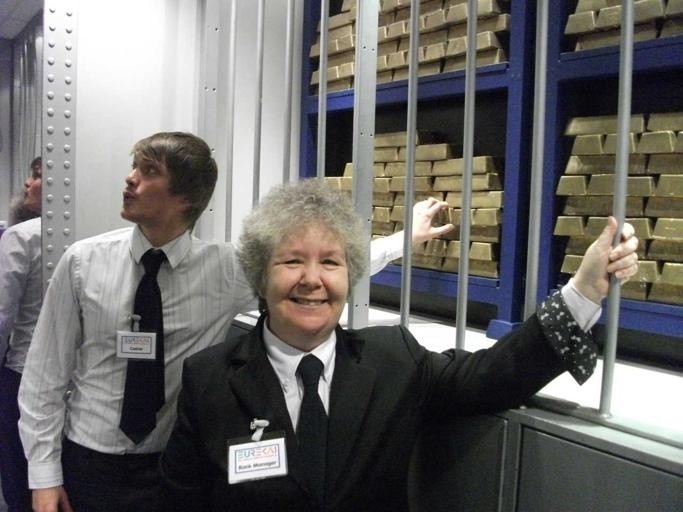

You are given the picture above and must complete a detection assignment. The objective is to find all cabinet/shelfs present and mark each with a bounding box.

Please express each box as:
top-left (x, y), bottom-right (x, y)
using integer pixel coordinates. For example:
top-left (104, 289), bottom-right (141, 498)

top-left (297, 1), bottom-right (528, 343)
top-left (230, 320), bottom-right (253, 340)
top-left (536, 1), bottom-right (682, 337)
top-left (434, 411), bottom-right (520, 512)
top-left (511, 411), bottom-right (681, 512)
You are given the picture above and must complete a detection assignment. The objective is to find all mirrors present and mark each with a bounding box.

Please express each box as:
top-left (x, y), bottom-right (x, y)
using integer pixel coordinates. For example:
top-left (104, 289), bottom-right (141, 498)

top-left (0, 0), bottom-right (75, 512)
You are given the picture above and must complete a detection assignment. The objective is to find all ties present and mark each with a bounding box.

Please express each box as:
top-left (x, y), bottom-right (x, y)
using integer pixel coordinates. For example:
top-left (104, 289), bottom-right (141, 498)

top-left (296, 354), bottom-right (329, 463)
top-left (119, 248), bottom-right (166, 446)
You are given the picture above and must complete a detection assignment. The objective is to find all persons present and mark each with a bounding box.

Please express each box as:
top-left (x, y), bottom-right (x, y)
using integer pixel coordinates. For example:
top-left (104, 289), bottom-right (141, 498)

top-left (0, 156), bottom-right (42, 511)
top-left (15, 131), bottom-right (458, 511)
top-left (159, 176), bottom-right (639, 511)
